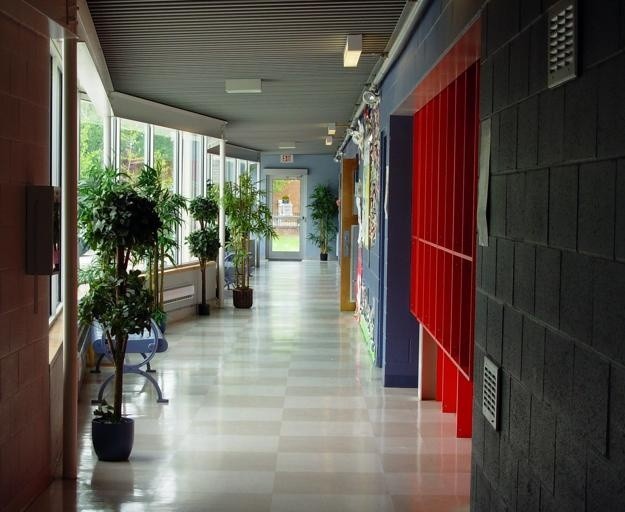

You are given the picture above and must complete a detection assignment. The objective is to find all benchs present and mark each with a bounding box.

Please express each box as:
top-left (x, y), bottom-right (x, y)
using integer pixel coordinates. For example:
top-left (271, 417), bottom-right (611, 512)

top-left (225, 249), bottom-right (255, 290)
top-left (91, 311), bottom-right (168, 405)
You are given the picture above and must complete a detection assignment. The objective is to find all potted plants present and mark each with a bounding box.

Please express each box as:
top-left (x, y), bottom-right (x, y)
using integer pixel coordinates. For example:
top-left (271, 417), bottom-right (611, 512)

top-left (213, 224), bottom-right (230, 299)
top-left (305, 185), bottom-right (338, 261)
top-left (186, 196), bottom-right (222, 316)
top-left (77, 161), bottom-right (162, 463)
top-left (208, 174), bottom-right (279, 308)
top-left (133, 149), bottom-right (188, 333)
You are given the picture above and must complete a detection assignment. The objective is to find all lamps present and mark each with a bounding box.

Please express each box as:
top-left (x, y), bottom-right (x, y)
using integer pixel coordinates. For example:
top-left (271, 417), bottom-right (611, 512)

top-left (346, 126), bottom-right (359, 136)
top-left (336, 151), bottom-right (344, 160)
top-left (328, 125), bottom-right (336, 135)
top-left (224, 79), bottom-right (261, 94)
top-left (363, 88), bottom-right (382, 105)
top-left (343, 34), bottom-right (362, 67)
top-left (326, 136), bottom-right (332, 145)
top-left (279, 142), bottom-right (296, 149)
